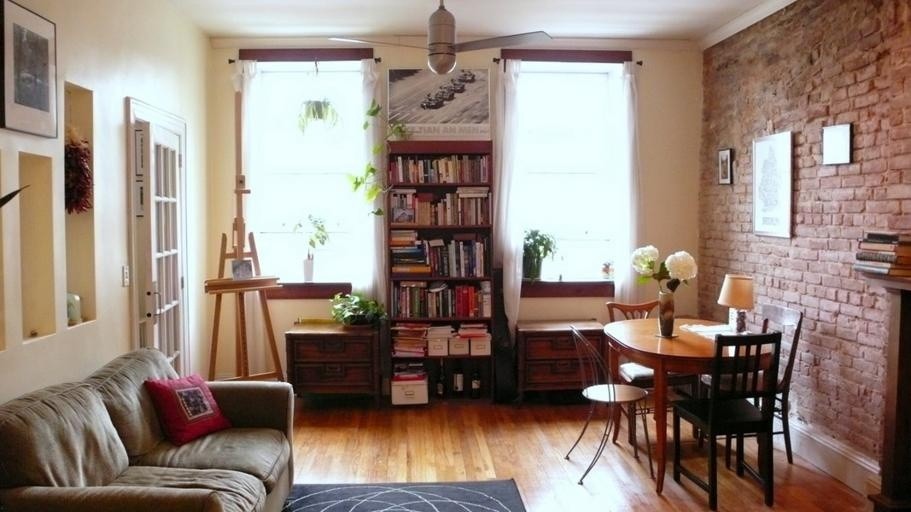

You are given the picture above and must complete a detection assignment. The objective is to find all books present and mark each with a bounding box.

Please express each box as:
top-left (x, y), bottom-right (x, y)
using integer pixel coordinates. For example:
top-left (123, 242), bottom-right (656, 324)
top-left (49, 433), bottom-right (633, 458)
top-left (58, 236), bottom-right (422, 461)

top-left (853, 232), bottom-right (911, 277)
top-left (387, 153), bottom-right (493, 358)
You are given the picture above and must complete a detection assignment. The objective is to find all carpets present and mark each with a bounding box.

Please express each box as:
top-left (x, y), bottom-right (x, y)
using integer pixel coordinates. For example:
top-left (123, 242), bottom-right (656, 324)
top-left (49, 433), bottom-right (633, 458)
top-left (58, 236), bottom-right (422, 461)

top-left (281, 478), bottom-right (526, 512)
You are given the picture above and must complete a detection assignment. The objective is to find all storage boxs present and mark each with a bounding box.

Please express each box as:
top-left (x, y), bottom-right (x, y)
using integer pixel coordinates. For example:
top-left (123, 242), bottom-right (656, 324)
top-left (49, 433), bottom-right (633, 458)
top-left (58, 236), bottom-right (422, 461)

top-left (391, 376), bottom-right (429, 406)
top-left (428, 337), bottom-right (491, 356)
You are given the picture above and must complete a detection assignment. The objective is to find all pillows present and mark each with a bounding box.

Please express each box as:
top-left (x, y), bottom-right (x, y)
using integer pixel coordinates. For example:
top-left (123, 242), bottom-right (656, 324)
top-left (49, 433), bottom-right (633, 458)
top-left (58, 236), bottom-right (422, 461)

top-left (1, 347), bottom-right (232, 487)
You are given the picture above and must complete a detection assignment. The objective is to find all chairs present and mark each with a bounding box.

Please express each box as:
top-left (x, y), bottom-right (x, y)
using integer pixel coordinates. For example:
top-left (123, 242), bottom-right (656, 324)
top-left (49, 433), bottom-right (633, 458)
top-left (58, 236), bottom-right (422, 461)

top-left (567, 324), bottom-right (656, 485)
top-left (608, 301), bottom-right (700, 445)
top-left (699, 303), bottom-right (803, 469)
top-left (672, 332), bottom-right (781, 511)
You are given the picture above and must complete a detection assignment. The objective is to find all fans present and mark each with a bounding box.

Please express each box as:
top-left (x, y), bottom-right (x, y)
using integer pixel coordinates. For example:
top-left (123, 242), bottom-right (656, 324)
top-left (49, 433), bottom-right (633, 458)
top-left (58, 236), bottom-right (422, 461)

top-left (329, 0), bottom-right (554, 75)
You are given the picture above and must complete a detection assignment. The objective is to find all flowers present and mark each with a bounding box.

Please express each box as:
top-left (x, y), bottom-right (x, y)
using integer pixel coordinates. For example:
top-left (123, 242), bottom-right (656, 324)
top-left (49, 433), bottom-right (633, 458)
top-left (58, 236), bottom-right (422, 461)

top-left (630, 244), bottom-right (698, 290)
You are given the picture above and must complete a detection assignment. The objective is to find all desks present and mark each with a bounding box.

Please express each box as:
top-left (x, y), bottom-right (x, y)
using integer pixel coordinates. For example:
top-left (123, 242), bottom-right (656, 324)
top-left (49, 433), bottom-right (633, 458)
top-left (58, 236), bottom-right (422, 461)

top-left (603, 316), bottom-right (774, 496)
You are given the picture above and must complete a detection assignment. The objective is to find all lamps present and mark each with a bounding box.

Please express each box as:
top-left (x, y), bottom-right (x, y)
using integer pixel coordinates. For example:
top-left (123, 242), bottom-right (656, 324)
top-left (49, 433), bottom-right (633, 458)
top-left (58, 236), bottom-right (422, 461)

top-left (718, 272), bottom-right (754, 331)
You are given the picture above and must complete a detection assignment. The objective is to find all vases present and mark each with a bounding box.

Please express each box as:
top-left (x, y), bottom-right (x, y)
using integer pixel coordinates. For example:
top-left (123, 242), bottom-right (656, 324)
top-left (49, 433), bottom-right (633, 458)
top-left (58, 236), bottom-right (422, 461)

top-left (657, 291), bottom-right (675, 336)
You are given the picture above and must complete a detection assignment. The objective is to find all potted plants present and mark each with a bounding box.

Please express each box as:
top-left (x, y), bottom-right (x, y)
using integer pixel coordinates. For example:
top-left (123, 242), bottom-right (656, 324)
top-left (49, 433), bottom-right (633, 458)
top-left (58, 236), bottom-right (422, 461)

top-left (329, 291), bottom-right (385, 328)
top-left (296, 211), bottom-right (326, 283)
top-left (523, 229), bottom-right (557, 281)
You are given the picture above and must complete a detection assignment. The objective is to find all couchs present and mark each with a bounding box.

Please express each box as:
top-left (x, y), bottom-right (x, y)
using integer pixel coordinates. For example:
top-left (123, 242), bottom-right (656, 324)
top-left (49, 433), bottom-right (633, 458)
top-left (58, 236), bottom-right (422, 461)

top-left (0, 345), bottom-right (294, 512)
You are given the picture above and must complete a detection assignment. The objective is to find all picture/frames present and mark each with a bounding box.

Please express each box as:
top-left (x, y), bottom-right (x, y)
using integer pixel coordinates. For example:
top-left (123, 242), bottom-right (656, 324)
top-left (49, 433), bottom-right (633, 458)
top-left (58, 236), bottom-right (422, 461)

top-left (0, 0), bottom-right (58, 139)
top-left (822, 124), bottom-right (851, 164)
top-left (135, 130), bottom-right (145, 177)
top-left (753, 131), bottom-right (792, 238)
top-left (718, 148), bottom-right (732, 185)
top-left (136, 180), bottom-right (144, 217)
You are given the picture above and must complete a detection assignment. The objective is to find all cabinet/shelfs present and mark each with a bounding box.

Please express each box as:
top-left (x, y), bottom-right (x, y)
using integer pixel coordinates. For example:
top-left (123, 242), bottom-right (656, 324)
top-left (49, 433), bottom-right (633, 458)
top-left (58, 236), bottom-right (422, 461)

top-left (516, 319), bottom-right (605, 406)
top-left (385, 139), bottom-right (493, 406)
top-left (285, 321), bottom-right (383, 409)
